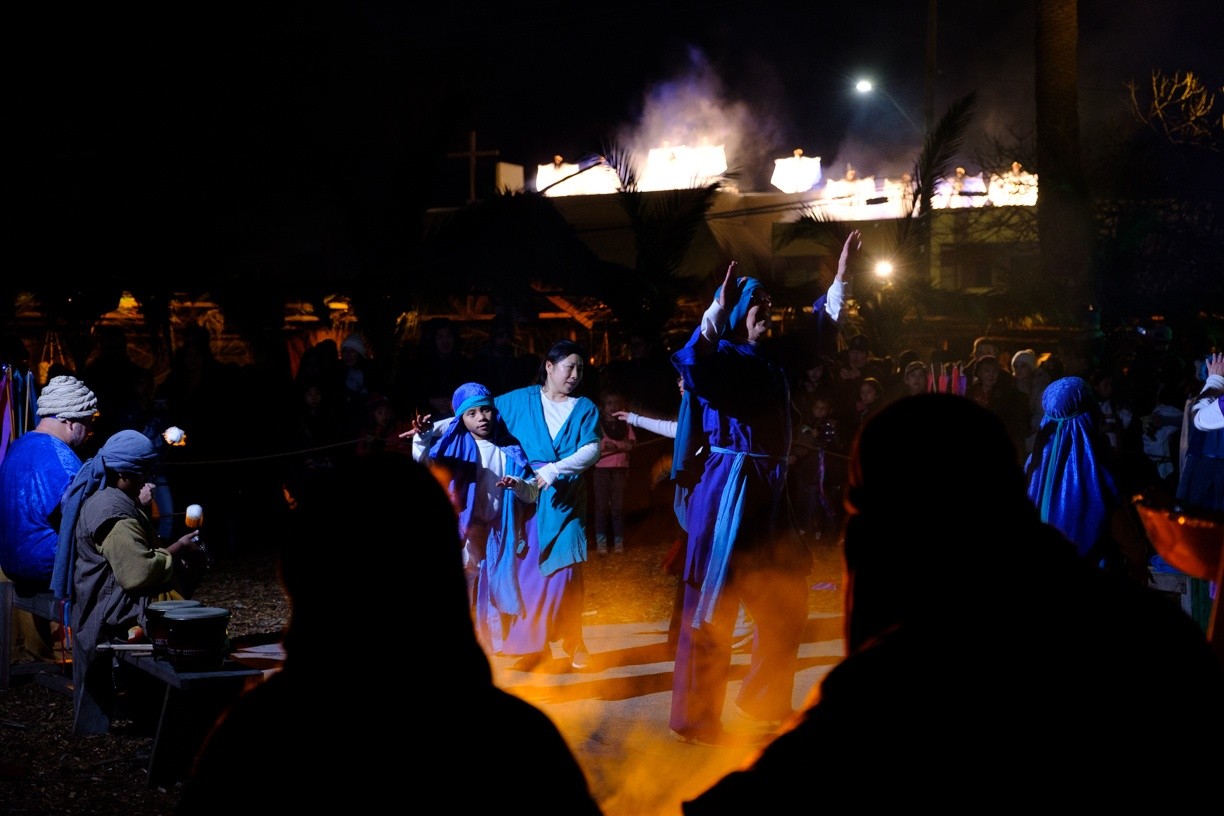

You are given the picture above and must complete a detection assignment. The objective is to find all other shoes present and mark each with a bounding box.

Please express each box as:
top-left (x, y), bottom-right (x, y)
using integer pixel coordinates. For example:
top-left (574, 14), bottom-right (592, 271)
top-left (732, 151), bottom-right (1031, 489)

top-left (669, 726), bottom-right (734, 749)
top-left (734, 705), bottom-right (800, 723)
top-left (561, 638), bottom-right (592, 668)
top-left (598, 549), bottom-right (608, 556)
top-left (616, 547), bottom-right (625, 553)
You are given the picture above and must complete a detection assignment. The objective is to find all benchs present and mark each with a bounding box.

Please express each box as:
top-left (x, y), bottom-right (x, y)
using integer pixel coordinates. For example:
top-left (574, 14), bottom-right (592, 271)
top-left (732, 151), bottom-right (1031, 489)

top-left (0, 581), bottom-right (263, 792)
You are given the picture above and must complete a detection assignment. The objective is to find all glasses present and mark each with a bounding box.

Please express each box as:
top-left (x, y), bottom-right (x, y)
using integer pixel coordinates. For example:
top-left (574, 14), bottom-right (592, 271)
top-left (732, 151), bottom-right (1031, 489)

top-left (750, 293), bottom-right (773, 305)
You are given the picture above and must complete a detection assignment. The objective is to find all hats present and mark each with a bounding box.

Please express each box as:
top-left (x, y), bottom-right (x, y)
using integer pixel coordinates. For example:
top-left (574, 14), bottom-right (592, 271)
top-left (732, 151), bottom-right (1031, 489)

top-left (36, 376), bottom-right (99, 420)
top-left (1011, 349), bottom-right (1036, 372)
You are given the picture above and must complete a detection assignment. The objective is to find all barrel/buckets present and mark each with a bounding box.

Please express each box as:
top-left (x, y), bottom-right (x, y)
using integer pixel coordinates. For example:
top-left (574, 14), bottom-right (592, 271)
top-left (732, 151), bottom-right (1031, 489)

top-left (163, 607), bottom-right (230, 673)
top-left (148, 600), bottom-right (201, 663)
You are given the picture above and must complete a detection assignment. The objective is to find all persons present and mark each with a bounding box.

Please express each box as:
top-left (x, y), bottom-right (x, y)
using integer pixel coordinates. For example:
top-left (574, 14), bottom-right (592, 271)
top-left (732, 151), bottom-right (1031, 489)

top-left (960, 332), bottom-right (1223, 642)
top-left (179, 445), bottom-right (601, 816)
top-left (785, 336), bottom-right (954, 545)
top-left (294, 315), bottom-right (679, 671)
top-left (0, 320), bottom-right (284, 737)
top-left (669, 231), bottom-right (870, 748)
top-left (679, 393), bottom-right (1223, 816)
